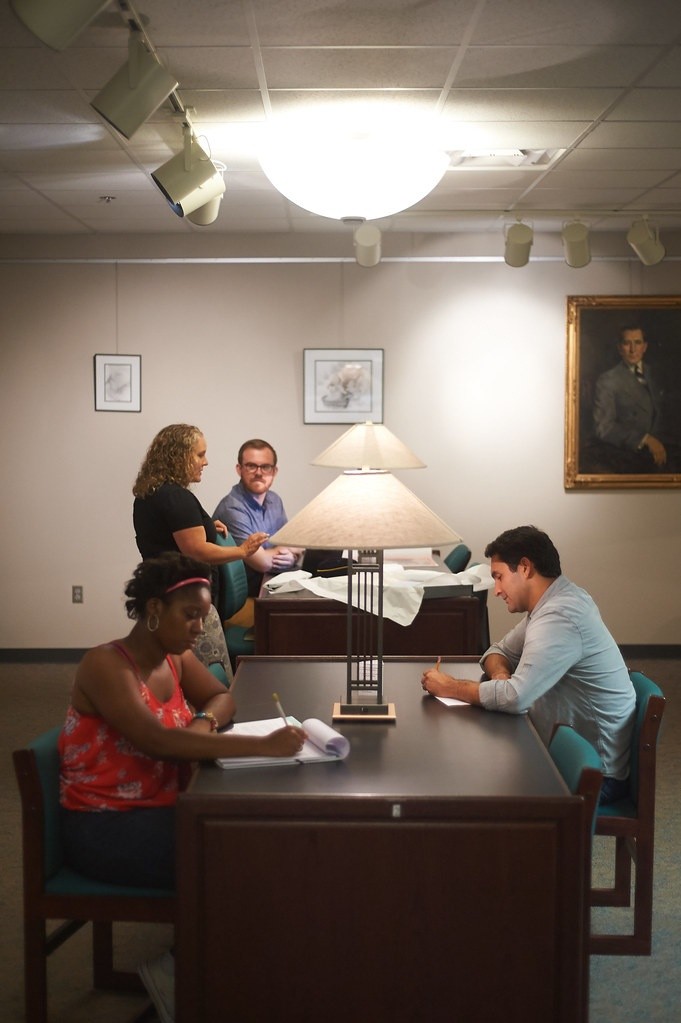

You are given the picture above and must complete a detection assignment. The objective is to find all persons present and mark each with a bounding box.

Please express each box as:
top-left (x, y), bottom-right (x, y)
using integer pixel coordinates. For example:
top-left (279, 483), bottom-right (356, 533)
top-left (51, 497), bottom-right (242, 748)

top-left (211, 438), bottom-right (308, 596)
top-left (59, 551), bottom-right (309, 1023)
top-left (421, 524), bottom-right (635, 803)
top-left (133, 424), bottom-right (271, 633)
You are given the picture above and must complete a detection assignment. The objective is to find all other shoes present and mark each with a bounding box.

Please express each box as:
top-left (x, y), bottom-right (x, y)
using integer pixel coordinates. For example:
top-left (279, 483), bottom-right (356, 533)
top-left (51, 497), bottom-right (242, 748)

top-left (138, 948), bottom-right (177, 1023)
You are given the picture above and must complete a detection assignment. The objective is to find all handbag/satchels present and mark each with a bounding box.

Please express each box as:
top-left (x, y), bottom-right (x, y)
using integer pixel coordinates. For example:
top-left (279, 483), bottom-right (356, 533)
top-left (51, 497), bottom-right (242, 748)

top-left (318, 558), bottom-right (357, 577)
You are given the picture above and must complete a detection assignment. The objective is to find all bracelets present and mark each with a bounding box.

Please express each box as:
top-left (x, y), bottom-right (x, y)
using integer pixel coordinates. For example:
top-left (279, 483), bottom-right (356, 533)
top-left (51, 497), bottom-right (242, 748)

top-left (191, 712), bottom-right (217, 735)
top-left (293, 554), bottom-right (298, 567)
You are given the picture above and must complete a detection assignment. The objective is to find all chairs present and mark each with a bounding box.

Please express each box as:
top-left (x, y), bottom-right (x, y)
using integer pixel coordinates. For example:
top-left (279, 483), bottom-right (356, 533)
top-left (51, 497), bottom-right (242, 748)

top-left (202, 661), bottom-right (232, 688)
top-left (548, 668), bottom-right (666, 956)
top-left (444, 544), bottom-right (491, 655)
top-left (214, 530), bottom-right (255, 655)
top-left (12, 725), bottom-right (177, 1023)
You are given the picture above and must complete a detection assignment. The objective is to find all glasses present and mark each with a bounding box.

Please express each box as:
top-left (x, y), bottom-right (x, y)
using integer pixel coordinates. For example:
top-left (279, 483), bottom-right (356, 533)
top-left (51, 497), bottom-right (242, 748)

top-left (241, 463), bottom-right (274, 475)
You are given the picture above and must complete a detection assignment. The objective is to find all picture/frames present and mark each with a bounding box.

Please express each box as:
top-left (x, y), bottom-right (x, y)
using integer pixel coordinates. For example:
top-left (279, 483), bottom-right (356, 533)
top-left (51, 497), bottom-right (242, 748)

top-left (563, 294), bottom-right (681, 490)
top-left (303, 348), bottom-right (384, 425)
top-left (94, 353), bottom-right (142, 413)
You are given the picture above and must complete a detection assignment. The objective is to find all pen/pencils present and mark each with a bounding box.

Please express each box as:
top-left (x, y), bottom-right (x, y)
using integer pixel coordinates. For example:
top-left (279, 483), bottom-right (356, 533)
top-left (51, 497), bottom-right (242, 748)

top-left (437, 657), bottom-right (441, 670)
top-left (273, 693), bottom-right (287, 725)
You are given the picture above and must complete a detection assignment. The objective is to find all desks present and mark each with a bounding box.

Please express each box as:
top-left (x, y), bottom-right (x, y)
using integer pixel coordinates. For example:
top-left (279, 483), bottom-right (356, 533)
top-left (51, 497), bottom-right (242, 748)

top-left (255, 574), bottom-right (480, 655)
top-left (175, 654), bottom-right (589, 1023)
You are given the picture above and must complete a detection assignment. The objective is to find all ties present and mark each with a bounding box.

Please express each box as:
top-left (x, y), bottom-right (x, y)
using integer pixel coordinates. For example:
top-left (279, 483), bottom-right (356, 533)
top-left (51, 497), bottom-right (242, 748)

top-left (635, 364), bottom-right (650, 392)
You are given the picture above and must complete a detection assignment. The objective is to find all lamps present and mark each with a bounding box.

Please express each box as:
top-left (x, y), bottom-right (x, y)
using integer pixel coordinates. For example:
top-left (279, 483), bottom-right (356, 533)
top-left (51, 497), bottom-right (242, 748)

top-left (268, 469), bottom-right (463, 722)
top-left (90, 30), bottom-right (179, 141)
top-left (152, 129), bottom-right (227, 226)
top-left (356, 226), bottom-right (382, 267)
top-left (503, 216), bottom-right (534, 267)
top-left (310, 425), bottom-right (428, 563)
top-left (560, 219), bottom-right (593, 268)
top-left (8, 0), bottom-right (115, 51)
top-left (259, 128), bottom-right (452, 221)
top-left (626, 215), bottom-right (666, 266)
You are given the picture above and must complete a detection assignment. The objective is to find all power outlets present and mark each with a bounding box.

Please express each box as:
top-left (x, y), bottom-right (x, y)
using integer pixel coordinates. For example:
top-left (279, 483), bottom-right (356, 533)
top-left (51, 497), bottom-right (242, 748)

top-left (72, 586), bottom-right (83, 603)
